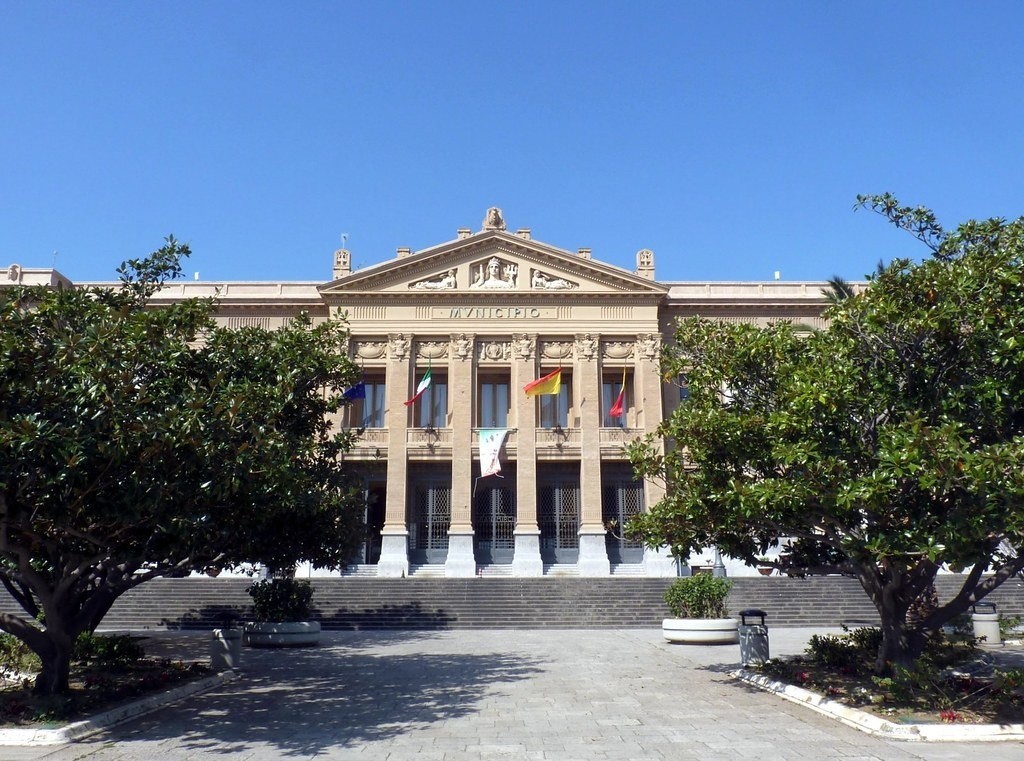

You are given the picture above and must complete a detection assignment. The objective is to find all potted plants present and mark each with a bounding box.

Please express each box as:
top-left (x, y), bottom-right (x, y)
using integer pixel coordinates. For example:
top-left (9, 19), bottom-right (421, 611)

top-left (662, 571), bottom-right (739, 643)
top-left (757, 558), bottom-right (774, 576)
top-left (244, 576), bottom-right (321, 645)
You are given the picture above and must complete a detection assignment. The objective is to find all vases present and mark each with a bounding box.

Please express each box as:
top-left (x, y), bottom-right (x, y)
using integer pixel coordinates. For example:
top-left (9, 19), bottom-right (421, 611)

top-left (207, 568), bottom-right (222, 577)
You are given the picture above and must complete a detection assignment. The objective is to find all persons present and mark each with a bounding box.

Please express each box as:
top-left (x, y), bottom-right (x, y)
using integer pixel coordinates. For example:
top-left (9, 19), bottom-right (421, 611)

top-left (415, 257), bottom-right (575, 291)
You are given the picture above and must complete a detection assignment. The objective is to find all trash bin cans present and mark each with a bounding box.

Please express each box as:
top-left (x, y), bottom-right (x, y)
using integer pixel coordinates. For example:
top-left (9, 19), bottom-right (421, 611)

top-left (210, 612), bottom-right (244, 671)
top-left (737, 608), bottom-right (770, 668)
top-left (971, 601), bottom-right (1004, 651)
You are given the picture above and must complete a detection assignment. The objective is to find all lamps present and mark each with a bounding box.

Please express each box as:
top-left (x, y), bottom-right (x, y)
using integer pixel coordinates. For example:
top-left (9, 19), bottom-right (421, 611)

top-left (424, 429), bottom-right (436, 447)
top-left (553, 425), bottom-right (563, 447)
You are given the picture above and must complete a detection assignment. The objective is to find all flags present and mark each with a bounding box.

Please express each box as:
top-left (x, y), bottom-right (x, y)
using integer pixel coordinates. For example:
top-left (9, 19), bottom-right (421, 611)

top-left (403, 358), bottom-right (433, 406)
top-left (478, 429), bottom-right (508, 478)
top-left (608, 357), bottom-right (626, 418)
top-left (522, 367), bottom-right (562, 399)
top-left (339, 370), bottom-right (365, 405)
